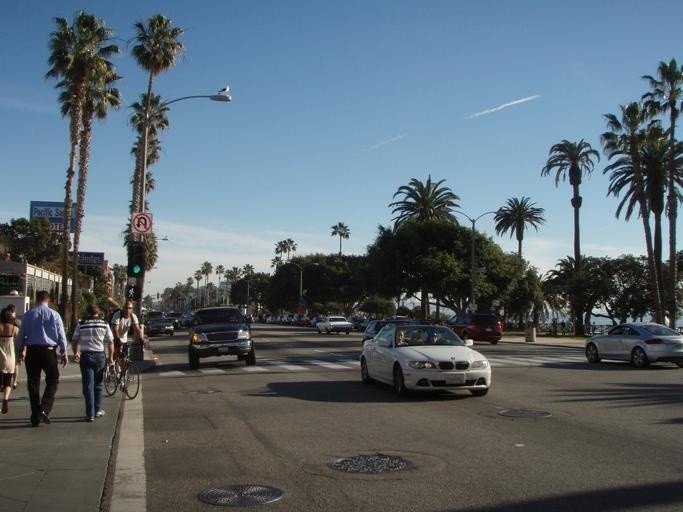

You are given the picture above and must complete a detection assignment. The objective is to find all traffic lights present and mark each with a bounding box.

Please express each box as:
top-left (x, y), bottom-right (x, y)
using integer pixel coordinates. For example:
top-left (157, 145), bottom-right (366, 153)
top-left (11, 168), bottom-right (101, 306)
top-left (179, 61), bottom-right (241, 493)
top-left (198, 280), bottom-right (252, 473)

top-left (129, 243), bottom-right (144, 276)
top-left (127, 286), bottom-right (135, 298)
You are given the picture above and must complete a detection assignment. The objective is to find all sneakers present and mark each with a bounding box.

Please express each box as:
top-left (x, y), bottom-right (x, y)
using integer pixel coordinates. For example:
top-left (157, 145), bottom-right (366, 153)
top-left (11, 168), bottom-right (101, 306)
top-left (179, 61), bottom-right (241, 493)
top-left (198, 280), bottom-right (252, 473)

top-left (96, 410), bottom-right (105, 416)
top-left (2, 398), bottom-right (8, 414)
top-left (86, 416), bottom-right (94, 421)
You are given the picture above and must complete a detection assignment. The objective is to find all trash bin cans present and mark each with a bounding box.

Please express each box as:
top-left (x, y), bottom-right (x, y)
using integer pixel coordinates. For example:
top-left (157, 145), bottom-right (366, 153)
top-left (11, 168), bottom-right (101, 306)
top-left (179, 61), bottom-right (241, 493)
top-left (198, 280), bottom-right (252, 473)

top-left (525, 323), bottom-right (536, 342)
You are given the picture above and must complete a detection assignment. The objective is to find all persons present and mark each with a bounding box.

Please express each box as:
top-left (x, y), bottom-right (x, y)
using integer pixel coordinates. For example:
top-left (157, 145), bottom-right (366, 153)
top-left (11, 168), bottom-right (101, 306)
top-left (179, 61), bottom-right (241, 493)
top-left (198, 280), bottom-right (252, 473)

top-left (560, 319), bottom-right (566, 336)
top-left (396, 329), bottom-right (408, 346)
top-left (589, 322), bottom-right (598, 337)
top-left (0, 308), bottom-right (19, 414)
top-left (426, 332), bottom-right (440, 344)
top-left (566, 318), bottom-right (573, 336)
top-left (70, 303), bottom-right (115, 422)
top-left (410, 330), bottom-right (423, 342)
top-left (13, 290), bottom-right (68, 427)
top-left (5, 304), bottom-right (22, 389)
top-left (106, 299), bottom-right (151, 378)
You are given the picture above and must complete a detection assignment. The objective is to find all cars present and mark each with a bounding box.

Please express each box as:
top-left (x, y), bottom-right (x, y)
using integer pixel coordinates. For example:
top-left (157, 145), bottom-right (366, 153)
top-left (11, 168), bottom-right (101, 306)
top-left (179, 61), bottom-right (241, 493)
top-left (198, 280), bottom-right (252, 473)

top-left (356, 326), bottom-right (491, 399)
top-left (147, 319), bottom-right (175, 336)
top-left (364, 320), bottom-right (414, 344)
top-left (582, 323), bottom-right (683, 371)
top-left (315, 315), bottom-right (353, 336)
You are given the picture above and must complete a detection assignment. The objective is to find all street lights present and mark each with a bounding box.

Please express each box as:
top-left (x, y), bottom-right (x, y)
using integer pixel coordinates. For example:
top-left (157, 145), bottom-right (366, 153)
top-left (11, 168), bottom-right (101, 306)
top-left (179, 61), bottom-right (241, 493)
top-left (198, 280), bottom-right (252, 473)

top-left (443, 205), bottom-right (509, 310)
top-left (134, 82), bottom-right (234, 325)
top-left (238, 277), bottom-right (261, 308)
top-left (287, 260), bottom-right (320, 307)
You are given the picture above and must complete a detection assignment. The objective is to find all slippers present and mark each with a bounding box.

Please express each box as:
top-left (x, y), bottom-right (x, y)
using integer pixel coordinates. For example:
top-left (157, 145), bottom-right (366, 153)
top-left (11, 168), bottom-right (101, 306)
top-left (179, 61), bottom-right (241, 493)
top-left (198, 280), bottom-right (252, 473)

top-left (12, 381), bottom-right (18, 389)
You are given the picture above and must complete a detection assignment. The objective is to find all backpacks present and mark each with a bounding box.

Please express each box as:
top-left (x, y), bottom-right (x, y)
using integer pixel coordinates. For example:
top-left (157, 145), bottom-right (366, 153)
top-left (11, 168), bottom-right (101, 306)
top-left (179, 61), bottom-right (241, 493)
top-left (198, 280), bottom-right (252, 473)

top-left (108, 308), bottom-right (133, 327)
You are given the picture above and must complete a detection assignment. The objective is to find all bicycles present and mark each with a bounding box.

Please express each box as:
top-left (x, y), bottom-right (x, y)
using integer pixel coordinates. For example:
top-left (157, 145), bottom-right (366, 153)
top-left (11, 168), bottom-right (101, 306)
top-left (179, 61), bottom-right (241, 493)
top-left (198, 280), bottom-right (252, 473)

top-left (101, 342), bottom-right (142, 400)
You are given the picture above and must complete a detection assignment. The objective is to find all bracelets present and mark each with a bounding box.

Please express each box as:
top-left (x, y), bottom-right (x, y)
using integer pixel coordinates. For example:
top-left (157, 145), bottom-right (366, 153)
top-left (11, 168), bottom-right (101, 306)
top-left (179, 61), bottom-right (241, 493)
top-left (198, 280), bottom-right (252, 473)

top-left (61, 353), bottom-right (67, 357)
top-left (139, 337), bottom-right (143, 341)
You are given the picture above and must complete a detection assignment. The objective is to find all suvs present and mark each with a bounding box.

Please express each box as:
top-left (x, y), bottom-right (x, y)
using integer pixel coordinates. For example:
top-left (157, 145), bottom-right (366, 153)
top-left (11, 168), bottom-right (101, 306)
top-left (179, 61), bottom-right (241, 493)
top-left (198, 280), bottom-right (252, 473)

top-left (167, 312), bottom-right (189, 329)
top-left (185, 308), bottom-right (257, 367)
top-left (148, 311), bottom-right (165, 320)
top-left (445, 314), bottom-right (502, 346)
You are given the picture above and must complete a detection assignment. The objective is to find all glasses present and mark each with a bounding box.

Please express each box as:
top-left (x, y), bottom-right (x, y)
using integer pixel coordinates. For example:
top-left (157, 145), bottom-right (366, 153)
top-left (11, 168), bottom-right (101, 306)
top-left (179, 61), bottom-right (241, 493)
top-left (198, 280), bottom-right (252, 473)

top-left (124, 306), bottom-right (132, 309)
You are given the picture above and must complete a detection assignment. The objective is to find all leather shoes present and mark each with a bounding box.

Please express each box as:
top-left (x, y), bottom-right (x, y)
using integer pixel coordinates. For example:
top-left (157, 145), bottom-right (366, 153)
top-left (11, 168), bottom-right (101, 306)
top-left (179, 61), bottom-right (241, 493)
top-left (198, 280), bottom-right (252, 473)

top-left (41, 411), bottom-right (50, 424)
